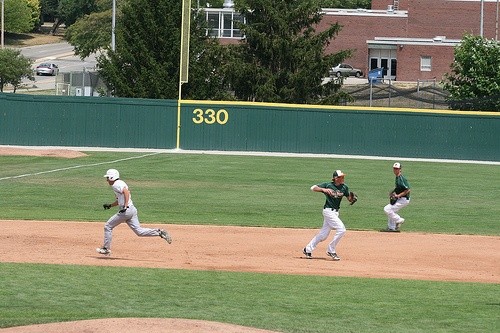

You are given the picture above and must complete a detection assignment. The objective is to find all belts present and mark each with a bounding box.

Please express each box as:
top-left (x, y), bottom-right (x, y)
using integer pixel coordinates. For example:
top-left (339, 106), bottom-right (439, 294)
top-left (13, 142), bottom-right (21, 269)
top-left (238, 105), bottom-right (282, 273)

top-left (399, 196), bottom-right (409, 200)
top-left (123, 206), bottom-right (129, 208)
top-left (324, 207), bottom-right (338, 212)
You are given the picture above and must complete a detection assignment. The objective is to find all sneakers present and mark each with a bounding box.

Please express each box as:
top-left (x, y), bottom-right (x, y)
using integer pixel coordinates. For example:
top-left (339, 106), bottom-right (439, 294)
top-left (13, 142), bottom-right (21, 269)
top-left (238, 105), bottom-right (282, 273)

top-left (302, 247), bottom-right (312, 258)
top-left (326, 252), bottom-right (341, 260)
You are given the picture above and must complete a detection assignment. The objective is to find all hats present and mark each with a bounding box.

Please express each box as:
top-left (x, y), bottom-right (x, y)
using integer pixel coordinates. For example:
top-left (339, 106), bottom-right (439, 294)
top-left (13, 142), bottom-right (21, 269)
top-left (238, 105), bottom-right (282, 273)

top-left (392, 162), bottom-right (401, 169)
top-left (333, 170), bottom-right (345, 177)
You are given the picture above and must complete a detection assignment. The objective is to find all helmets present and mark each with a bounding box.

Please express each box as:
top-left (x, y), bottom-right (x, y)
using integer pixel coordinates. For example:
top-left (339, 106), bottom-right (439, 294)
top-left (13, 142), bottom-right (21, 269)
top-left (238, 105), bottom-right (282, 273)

top-left (104, 169), bottom-right (119, 182)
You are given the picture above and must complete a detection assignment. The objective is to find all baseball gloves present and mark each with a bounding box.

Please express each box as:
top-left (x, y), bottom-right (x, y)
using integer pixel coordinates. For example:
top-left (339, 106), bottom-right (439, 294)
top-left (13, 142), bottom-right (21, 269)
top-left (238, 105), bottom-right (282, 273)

top-left (390, 194), bottom-right (398, 204)
top-left (348, 192), bottom-right (358, 204)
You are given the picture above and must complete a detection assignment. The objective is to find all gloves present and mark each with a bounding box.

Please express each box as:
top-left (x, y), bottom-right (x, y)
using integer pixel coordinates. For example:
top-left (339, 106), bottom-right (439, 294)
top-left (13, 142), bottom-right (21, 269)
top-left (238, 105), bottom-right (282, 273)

top-left (119, 208), bottom-right (127, 213)
top-left (103, 204), bottom-right (111, 209)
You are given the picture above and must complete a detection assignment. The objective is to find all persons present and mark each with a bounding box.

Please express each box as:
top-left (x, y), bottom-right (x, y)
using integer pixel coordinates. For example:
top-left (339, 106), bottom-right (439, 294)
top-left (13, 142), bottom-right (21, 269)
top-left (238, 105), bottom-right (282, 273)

top-left (383, 163), bottom-right (410, 230)
top-left (302, 170), bottom-right (357, 261)
top-left (95, 169), bottom-right (172, 254)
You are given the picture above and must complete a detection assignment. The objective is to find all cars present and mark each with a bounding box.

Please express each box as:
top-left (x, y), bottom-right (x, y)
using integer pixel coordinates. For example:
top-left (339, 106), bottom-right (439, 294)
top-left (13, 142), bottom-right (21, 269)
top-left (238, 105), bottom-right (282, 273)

top-left (329, 63), bottom-right (363, 78)
top-left (36, 62), bottom-right (59, 76)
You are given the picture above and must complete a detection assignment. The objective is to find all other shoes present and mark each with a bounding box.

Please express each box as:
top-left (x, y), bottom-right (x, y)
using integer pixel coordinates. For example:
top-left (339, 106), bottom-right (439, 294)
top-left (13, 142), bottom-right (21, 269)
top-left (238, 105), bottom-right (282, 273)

top-left (397, 218), bottom-right (404, 227)
top-left (385, 228), bottom-right (397, 231)
top-left (96, 247), bottom-right (111, 254)
top-left (160, 230), bottom-right (172, 244)
